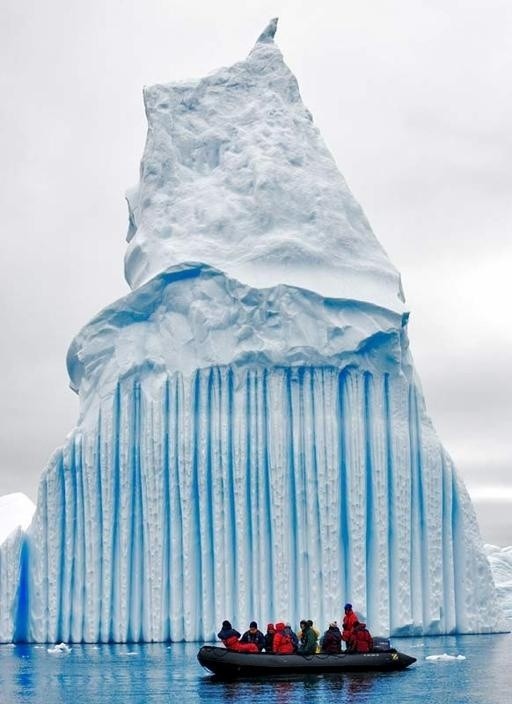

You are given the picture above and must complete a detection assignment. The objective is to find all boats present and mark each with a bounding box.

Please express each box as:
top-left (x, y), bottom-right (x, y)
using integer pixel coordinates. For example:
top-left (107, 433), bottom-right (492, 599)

top-left (198, 646), bottom-right (417, 682)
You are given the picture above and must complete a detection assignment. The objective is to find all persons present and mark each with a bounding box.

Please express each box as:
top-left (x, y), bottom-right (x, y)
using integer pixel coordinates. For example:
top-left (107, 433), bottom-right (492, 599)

top-left (217, 603), bottom-right (375, 650)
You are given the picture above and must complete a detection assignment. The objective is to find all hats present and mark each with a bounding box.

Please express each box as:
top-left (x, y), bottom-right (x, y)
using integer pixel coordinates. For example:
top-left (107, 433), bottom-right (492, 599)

top-left (250, 622), bottom-right (257, 628)
top-left (223, 621), bottom-right (231, 629)
top-left (268, 624), bottom-right (273, 629)
top-left (344, 604), bottom-right (352, 608)
top-left (276, 623), bottom-right (285, 631)
top-left (331, 621), bottom-right (337, 627)
